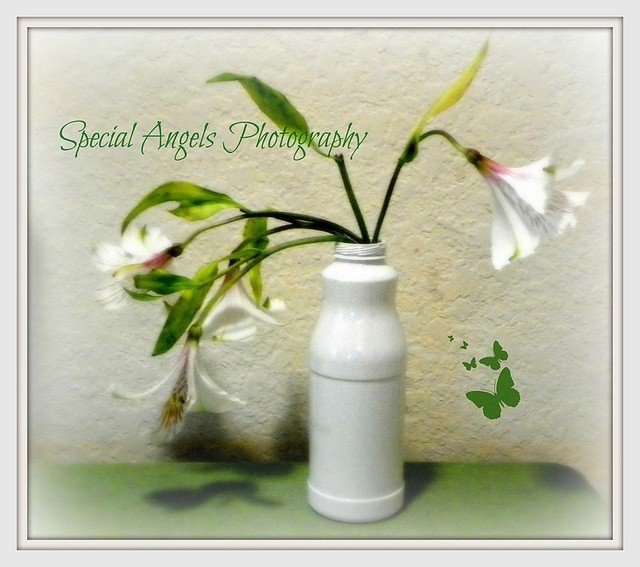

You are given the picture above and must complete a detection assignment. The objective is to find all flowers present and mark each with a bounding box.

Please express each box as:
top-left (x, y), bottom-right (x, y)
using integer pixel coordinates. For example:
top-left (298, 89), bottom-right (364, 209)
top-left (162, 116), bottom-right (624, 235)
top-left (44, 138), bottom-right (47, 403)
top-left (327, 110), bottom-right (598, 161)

top-left (94, 36), bottom-right (589, 446)
